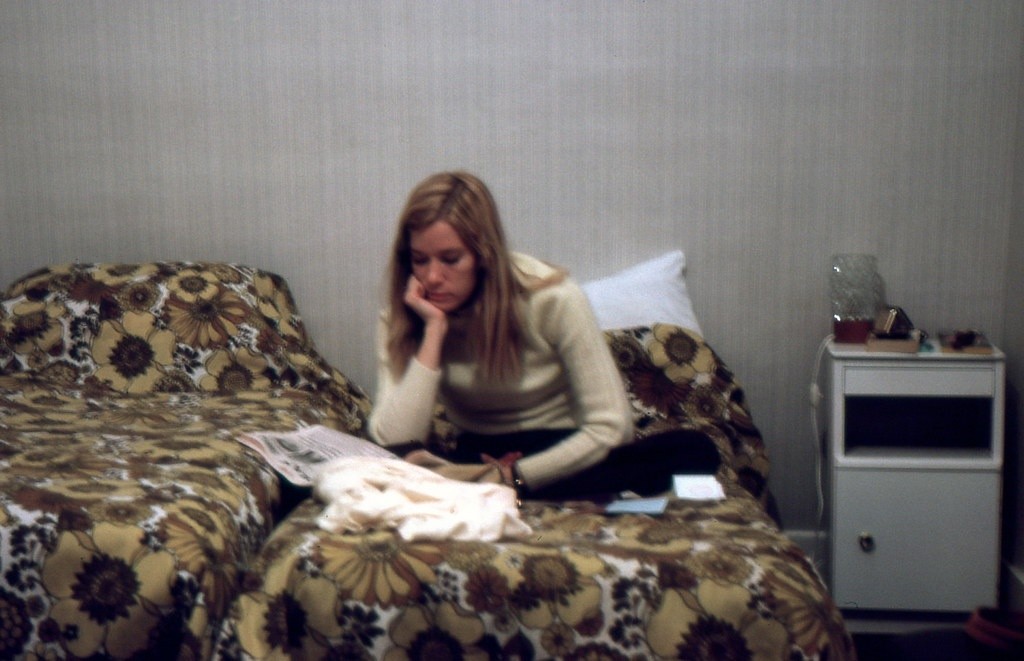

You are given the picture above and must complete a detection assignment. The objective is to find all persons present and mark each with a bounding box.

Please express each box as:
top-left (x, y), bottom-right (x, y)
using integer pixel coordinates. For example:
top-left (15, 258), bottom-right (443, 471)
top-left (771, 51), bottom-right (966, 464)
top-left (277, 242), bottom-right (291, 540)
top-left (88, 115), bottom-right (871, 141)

top-left (366, 172), bottom-right (721, 506)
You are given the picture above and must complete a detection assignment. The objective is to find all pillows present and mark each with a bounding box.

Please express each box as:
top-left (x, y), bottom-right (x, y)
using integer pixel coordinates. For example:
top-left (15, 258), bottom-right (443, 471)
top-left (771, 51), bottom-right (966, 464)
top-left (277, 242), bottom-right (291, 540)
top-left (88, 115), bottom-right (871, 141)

top-left (580, 249), bottom-right (704, 337)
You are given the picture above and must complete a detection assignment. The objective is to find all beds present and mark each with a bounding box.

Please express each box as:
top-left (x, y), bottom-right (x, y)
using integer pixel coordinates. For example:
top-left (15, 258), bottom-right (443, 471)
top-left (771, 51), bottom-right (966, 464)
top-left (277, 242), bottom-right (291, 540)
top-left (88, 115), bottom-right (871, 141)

top-left (0, 259), bottom-right (374, 661)
top-left (213, 323), bottom-right (857, 661)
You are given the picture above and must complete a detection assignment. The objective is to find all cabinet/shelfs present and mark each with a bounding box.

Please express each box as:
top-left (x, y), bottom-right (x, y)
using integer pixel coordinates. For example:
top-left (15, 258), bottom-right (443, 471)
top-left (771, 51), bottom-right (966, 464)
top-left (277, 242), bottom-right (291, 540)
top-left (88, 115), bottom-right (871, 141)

top-left (825, 338), bottom-right (1006, 635)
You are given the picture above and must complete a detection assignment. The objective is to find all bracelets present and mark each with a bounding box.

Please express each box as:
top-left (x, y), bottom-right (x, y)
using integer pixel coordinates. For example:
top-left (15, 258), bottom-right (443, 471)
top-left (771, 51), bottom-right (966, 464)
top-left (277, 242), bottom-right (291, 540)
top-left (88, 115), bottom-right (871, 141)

top-left (511, 470), bottom-right (524, 507)
